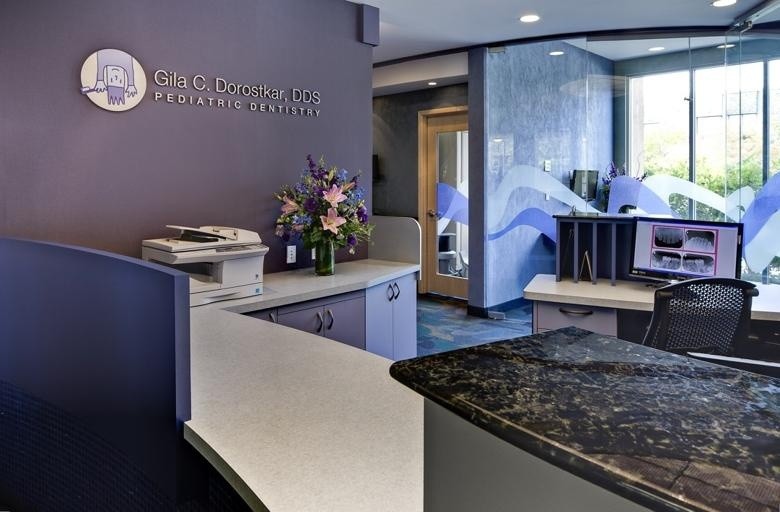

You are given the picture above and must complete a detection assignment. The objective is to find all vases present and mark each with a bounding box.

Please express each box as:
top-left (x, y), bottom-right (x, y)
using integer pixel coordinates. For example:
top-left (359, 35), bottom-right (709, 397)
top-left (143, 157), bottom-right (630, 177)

top-left (313, 235), bottom-right (335, 274)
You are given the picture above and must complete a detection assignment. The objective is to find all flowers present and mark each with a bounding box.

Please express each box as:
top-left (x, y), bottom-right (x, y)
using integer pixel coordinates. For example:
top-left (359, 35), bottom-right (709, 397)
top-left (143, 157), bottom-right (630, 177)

top-left (597, 164), bottom-right (648, 207)
top-left (271, 152), bottom-right (377, 272)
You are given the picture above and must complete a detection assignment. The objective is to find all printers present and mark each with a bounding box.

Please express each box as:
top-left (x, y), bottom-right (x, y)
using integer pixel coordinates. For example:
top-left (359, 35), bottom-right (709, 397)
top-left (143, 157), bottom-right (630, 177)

top-left (143, 224), bottom-right (269, 308)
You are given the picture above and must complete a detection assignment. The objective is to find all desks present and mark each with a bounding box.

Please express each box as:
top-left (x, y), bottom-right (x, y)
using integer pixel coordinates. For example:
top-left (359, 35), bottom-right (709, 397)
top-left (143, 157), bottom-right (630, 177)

top-left (435, 233), bottom-right (456, 262)
top-left (183, 255), bottom-right (425, 512)
top-left (524, 211), bottom-right (779, 339)
top-left (388, 325), bottom-right (780, 512)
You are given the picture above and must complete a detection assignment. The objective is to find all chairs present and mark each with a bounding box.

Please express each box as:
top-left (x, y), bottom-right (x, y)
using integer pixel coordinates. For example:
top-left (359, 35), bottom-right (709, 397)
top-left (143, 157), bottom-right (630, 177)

top-left (448, 253), bottom-right (468, 279)
top-left (641, 278), bottom-right (759, 363)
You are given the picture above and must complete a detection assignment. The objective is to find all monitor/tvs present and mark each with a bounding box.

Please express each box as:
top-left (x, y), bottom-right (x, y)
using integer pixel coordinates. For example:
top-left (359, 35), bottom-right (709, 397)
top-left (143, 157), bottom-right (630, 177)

top-left (570, 169), bottom-right (598, 201)
top-left (627, 217), bottom-right (744, 301)
